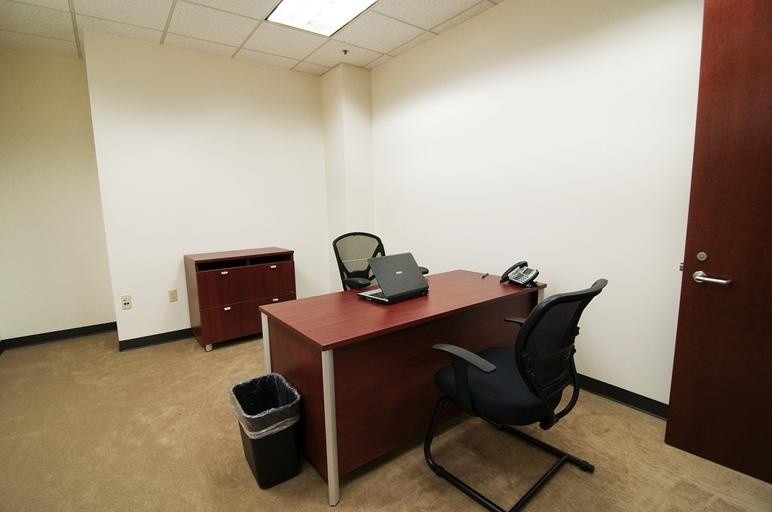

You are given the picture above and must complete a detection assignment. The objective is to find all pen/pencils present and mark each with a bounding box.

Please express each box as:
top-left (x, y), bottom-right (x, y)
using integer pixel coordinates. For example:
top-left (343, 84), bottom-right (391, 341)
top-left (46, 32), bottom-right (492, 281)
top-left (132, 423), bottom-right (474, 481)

top-left (482, 273), bottom-right (489, 279)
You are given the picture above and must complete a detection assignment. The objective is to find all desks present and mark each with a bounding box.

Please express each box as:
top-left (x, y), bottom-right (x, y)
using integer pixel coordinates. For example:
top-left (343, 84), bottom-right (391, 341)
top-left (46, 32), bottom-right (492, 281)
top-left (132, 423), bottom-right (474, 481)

top-left (258, 269), bottom-right (547, 507)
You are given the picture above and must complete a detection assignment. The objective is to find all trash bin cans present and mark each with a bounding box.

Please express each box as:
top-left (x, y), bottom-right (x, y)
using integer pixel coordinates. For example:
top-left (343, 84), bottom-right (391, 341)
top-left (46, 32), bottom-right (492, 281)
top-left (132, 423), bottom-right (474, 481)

top-left (231, 373), bottom-right (302, 490)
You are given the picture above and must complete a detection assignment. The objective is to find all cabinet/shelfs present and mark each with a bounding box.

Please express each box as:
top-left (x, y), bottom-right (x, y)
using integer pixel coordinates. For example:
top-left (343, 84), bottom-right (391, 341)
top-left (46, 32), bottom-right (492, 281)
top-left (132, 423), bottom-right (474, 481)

top-left (184, 247), bottom-right (296, 352)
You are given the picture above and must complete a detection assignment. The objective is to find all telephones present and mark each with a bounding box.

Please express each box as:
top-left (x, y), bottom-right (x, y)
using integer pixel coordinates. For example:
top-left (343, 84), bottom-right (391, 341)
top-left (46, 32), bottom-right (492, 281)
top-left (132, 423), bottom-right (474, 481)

top-left (501, 261), bottom-right (540, 288)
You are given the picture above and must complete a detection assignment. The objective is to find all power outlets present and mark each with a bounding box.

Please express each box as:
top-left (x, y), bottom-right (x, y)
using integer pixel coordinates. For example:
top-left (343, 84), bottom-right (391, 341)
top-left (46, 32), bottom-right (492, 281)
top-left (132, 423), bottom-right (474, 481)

top-left (121, 295), bottom-right (131, 310)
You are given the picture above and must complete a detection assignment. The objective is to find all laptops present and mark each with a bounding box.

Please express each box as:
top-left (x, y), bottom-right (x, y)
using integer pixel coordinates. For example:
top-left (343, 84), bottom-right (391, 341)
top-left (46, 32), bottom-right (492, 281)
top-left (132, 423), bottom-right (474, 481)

top-left (355, 252), bottom-right (430, 305)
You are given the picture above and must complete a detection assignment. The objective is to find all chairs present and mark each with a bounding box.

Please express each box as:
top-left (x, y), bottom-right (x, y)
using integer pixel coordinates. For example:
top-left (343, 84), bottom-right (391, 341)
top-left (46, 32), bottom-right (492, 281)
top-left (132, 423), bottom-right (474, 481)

top-left (423, 278), bottom-right (609, 512)
top-left (333, 232), bottom-right (428, 291)
